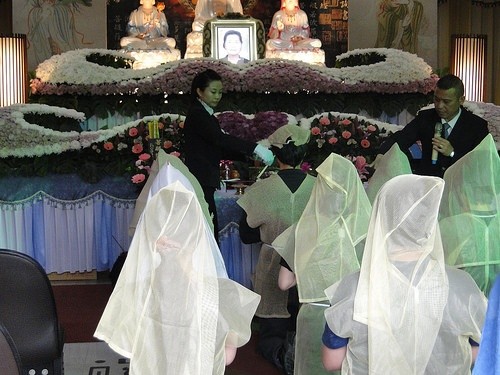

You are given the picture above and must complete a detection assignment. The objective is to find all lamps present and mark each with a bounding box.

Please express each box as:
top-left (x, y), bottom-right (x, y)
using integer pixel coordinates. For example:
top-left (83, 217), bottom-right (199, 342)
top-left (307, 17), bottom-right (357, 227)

top-left (0, 33), bottom-right (27, 109)
top-left (451, 34), bottom-right (488, 102)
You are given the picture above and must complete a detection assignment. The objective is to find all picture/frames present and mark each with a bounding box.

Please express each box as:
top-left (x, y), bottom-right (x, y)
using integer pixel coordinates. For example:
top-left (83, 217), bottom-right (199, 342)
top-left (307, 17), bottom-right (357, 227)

top-left (209, 19), bottom-right (258, 65)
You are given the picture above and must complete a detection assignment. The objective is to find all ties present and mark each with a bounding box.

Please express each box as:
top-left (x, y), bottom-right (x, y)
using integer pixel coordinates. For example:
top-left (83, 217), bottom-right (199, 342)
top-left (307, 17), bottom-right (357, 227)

top-left (436, 123), bottom-right (451, 164)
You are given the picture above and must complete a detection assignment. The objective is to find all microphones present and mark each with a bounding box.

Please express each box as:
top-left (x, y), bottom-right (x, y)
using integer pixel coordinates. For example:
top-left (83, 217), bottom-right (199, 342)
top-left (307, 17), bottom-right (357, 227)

top-left (432, 121), bottom-right (443, 164)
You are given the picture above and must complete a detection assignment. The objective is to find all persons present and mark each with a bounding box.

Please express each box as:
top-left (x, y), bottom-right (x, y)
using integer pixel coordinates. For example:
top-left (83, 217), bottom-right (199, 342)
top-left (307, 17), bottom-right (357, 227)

top-left (222, 31), bottom-right (248, 64)
top-left (192, 0), bottom-right (243, 31)
top-left (266, 0), bottom-right (321, 51)
top-left (93, 149), bottom-right (261, 375)
top-left (184, 69), bottom-right (274, 243)
top-left (237, 125), bottom-right (500, 375)
top-left (378, 74), bottom-right (489, 179)
top-left (120, 0), bottom-right (176, 50)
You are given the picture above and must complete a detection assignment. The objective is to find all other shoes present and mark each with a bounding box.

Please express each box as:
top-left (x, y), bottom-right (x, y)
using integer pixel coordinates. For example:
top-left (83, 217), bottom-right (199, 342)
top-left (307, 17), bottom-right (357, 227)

top-left (283, 331), bottom-right (296, 375)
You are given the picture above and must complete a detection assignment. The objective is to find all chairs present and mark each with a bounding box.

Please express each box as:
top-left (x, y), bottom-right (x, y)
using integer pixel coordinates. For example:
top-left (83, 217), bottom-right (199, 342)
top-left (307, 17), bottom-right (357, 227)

top-left (0, 248), bottom-right (64, 374)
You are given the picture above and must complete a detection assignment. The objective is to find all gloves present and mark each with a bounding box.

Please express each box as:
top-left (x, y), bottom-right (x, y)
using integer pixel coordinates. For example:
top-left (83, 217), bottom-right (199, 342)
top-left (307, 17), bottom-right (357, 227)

top-left (254, 144), bottom-right (274, 166)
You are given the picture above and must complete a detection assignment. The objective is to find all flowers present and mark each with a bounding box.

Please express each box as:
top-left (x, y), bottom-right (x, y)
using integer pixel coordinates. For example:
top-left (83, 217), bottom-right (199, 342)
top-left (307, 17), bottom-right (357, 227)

top-left (0, 44), bottom-right (500, 184)
